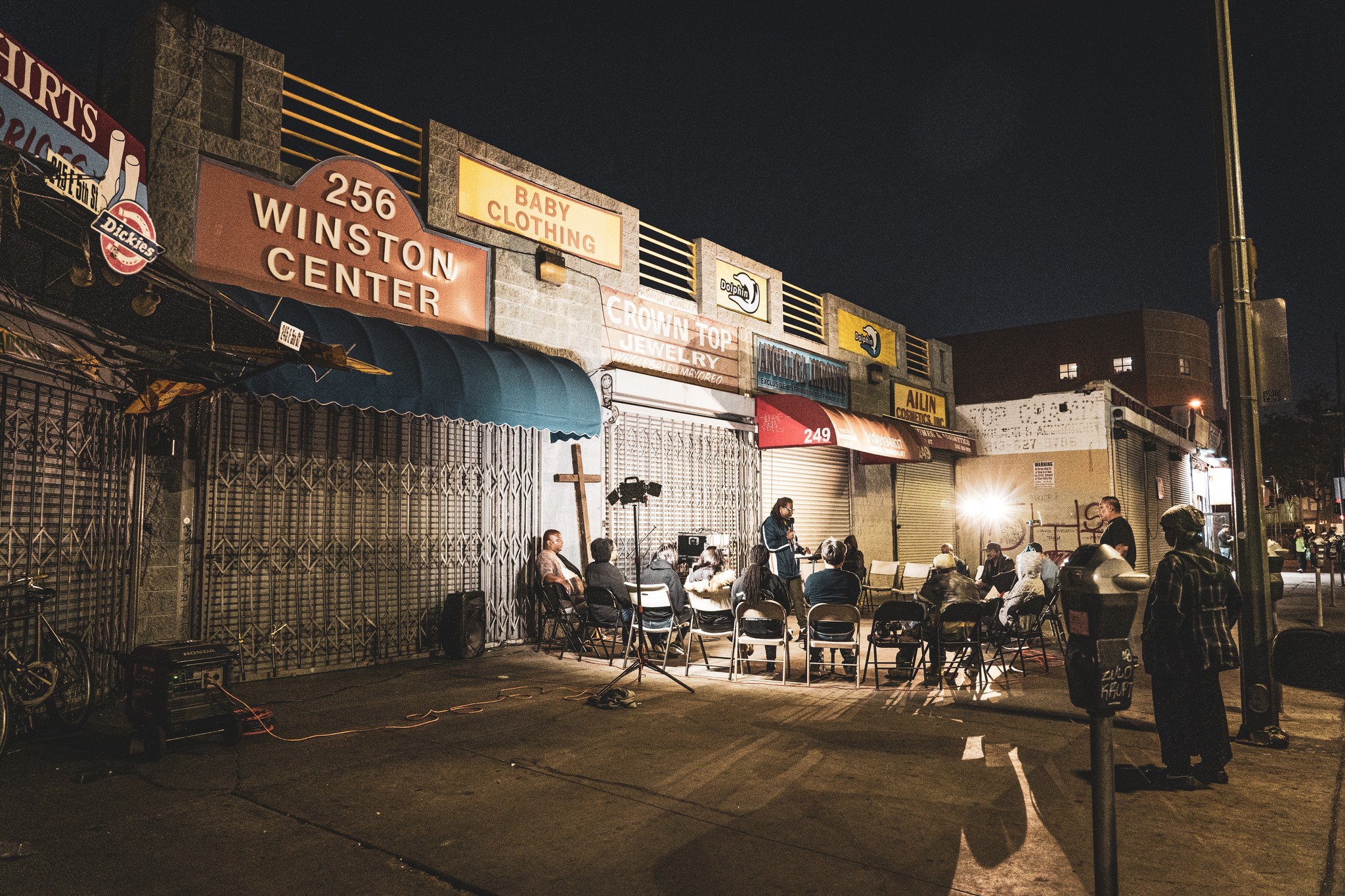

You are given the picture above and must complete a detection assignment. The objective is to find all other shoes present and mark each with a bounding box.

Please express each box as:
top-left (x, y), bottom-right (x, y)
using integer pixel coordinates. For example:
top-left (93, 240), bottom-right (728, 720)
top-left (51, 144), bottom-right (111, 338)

top-left (960, 654), bottom-right (981, 673)
top-left (787, 627), bottom-right (793, 640)
top-left (798, 629), bottom-right (808, 640)
top-left (567, 643), bottom-right (593, 653)
top-left (809, 654), bottom-right (823, 676)
top-left (747, 644), bottom-right (754, 656)
top-left (842, 660), bottom-right (857, 678)
top-left (1191, 763), bottom-right (1229, 783)
top-left (1297, 569), bottom-right (1303, 573)
top-left (1145, 770), bottom-right (1197, 791)
top-left (888, 669), bottom-right (911, 677)
top-left (621, 649), bottom-right (637, 657)
top-left (926, 665), bottom-right (944, 675)
top-left (669, 641), bottom-right (684, 655)
top-left (1002, 640), bottom-right (1029, 649)
top-left (766, 664), bottom-right (776, 673)
top-left (734, 660), bottom-right (741, 666)
top-left (654, 640), bottom-right (668, 658)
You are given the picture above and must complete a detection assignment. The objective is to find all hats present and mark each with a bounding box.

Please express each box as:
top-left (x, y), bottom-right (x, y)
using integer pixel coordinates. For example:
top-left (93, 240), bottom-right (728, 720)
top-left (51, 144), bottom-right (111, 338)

top-left (982, 542), bottom-right (1001, 552)
top-left (1295, 529), bottom-right (1305, 534)
top-left (929, 553), bottom-right (956, 570)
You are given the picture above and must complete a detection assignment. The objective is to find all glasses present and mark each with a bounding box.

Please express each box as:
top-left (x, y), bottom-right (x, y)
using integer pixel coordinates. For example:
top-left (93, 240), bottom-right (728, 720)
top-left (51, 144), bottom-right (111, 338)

top-left (784, 506), bottom-right (795, 512)
top-left (612, 547), bottom-right (618, 551)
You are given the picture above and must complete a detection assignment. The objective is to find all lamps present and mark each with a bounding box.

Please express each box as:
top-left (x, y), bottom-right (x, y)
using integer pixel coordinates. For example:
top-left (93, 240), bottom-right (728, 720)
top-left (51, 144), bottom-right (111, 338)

top-left (536, 246), bottom-right (567, 287)
top-left (99, 260), bottom-right (124, 287)
top-left (131, 282), bottom-right (161, 316)
top-left (867, 363), bottom-right (884, 386)
top-left (71, 235), bottom-right (96, 287)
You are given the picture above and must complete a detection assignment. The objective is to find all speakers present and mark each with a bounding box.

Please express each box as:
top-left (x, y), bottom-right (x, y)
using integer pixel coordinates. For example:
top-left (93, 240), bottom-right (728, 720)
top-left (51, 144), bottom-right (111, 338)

top-left (443, 591), bottom-right (487, 658)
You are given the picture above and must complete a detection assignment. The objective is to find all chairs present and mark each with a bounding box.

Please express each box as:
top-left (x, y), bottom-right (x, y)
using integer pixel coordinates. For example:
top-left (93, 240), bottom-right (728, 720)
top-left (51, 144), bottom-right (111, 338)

top-left (535, 560), bottom-right (1067, 692)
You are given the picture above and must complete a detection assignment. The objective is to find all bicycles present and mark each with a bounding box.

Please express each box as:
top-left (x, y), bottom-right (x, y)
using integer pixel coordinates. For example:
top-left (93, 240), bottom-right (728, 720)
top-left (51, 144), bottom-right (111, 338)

top-left (0, 574), bottom-right (95, 757)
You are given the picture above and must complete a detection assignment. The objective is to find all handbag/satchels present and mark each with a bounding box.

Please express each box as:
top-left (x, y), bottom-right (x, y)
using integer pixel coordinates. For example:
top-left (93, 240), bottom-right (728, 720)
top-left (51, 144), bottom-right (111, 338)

top-left (1306, 548), bottom-right (1310, 560)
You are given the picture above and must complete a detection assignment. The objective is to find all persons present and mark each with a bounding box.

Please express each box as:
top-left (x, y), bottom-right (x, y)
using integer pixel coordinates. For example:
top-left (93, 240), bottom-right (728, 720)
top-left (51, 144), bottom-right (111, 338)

top-left (1289, 524), bottom-right (1345, 573)
top-left (1217, 524), bottom-right (1234, 573)
top-left (683, 546), bottom-right (754, 666)
top-left (760, 497), bottom-right (811, 641)
top-left (535, 529), bottom-right (597, 653)
top-left (1002, 542), bottom-right (1060, 650)
top-left (585, 538), bottom-right (639, 657)
top-left (640, 542), bottom-right (694, 659)
top-left (931, 542), bottom-right (971, 578)
top-left (1141, 504), bottom-right (1244, 791)
top-left (959, 551), bottom-right (1044, 673)
top-left (730, 544), bottom-right (792, 673)
top-left (1096, 496), bottom-right (1139, 668)
top-left (976, 542), bottom-right (1018, 600)
top-left (888, 553), bottom-right (980, 680)
top-left (803, 538), bottom-right (862, 678)
top-left (841, 535), bottom-right (864, 608)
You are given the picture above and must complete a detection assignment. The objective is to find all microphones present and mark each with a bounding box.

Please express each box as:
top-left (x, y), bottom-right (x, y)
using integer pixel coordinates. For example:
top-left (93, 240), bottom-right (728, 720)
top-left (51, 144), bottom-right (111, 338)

top-left (1026, 520), bottom-right (1039, 524)
top-left (654, 526), bottom-right (657, 528)
top-left (789, 517), bottom-right (795, 532)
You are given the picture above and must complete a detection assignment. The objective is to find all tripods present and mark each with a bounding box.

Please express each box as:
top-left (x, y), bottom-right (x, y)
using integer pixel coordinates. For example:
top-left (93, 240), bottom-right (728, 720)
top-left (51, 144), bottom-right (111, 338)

top-left (597, 505), bottom-right (696, 695)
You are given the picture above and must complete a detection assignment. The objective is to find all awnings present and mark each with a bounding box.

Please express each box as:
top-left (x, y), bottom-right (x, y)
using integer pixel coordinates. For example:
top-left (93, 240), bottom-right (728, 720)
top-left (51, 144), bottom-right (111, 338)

top-left (184, 280), bottom-right (601, 443)
top-left (755, 393), bottom-right (933, 466)
top-left (18, 179), bottom-right (393, 399)
